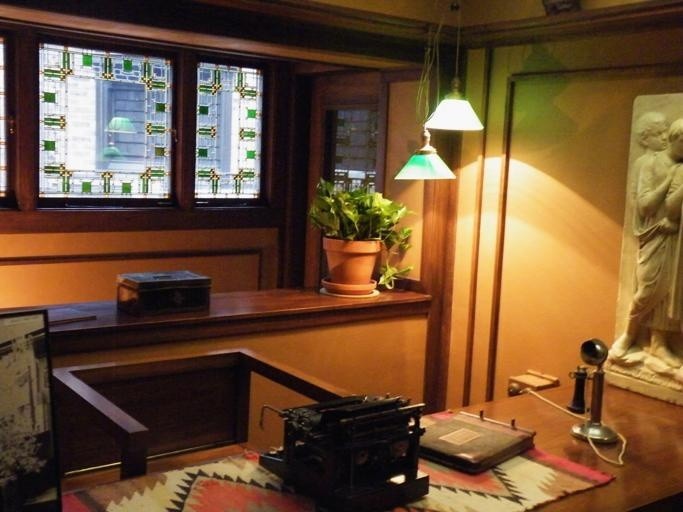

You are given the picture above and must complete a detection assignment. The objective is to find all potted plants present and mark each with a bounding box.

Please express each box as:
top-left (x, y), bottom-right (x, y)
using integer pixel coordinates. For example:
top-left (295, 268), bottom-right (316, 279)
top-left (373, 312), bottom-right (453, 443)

top-left (312, 175), bottom-right (409, 292)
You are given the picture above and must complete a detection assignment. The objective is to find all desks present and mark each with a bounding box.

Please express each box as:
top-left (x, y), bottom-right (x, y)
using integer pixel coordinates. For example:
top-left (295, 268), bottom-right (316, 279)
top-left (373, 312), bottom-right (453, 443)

top-left (1, 379), bottom-right (682, 510)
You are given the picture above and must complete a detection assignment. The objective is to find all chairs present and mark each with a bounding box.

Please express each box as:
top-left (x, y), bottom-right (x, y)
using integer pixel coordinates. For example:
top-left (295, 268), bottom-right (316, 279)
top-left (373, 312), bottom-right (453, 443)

top-left (49, 346), bottom-right (354, 495)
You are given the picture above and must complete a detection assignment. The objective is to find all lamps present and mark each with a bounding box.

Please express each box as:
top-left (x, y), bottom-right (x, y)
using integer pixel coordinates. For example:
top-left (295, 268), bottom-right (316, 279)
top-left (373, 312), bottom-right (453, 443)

top-left (390, 0), bottom-right (484, 182)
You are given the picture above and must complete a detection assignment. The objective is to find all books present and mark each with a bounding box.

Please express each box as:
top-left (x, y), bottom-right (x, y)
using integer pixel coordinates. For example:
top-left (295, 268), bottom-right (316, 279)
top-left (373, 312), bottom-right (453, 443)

top-left (408, 407), bottom-right (537, 475)
